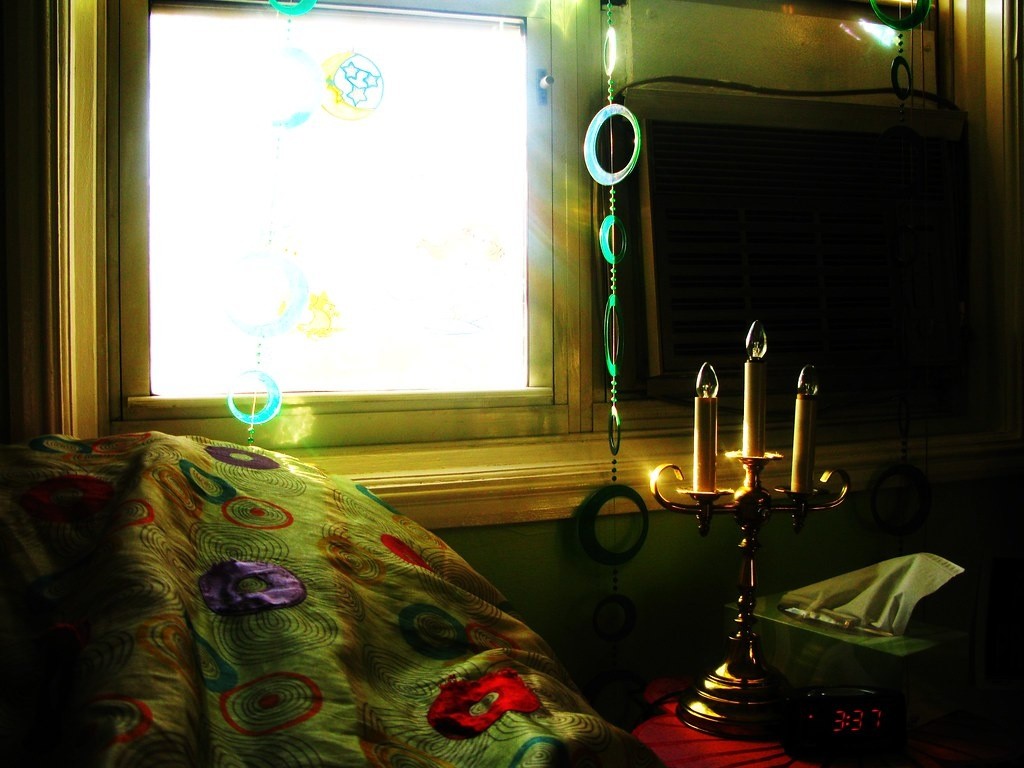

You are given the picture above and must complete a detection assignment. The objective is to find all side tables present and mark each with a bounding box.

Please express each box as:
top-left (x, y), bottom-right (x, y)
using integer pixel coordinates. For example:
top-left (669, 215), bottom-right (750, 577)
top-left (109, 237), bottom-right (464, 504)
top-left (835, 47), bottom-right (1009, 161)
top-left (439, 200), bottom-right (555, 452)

top-left (631, 692), bottom-right (1024, 768)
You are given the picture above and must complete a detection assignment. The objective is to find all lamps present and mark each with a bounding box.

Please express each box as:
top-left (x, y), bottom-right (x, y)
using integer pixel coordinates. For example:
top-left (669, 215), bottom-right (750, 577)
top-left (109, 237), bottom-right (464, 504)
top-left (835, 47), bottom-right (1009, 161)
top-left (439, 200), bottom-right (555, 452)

top-left (650, 320), bottom-right (851, 736)
